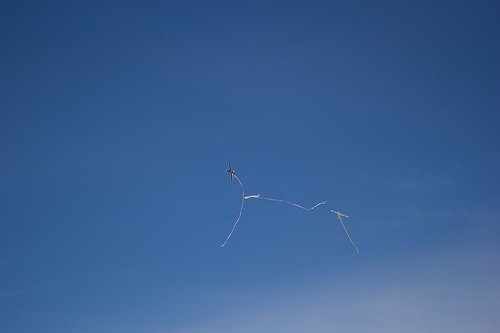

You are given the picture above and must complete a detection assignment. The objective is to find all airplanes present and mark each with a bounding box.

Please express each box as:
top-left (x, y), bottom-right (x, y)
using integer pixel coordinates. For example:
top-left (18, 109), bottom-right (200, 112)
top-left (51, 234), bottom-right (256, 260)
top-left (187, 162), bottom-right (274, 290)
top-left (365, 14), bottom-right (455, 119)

top-left (226, 161), bottom-right (235, 182)
top-left (331, 210), bottom-right (349, 219)
